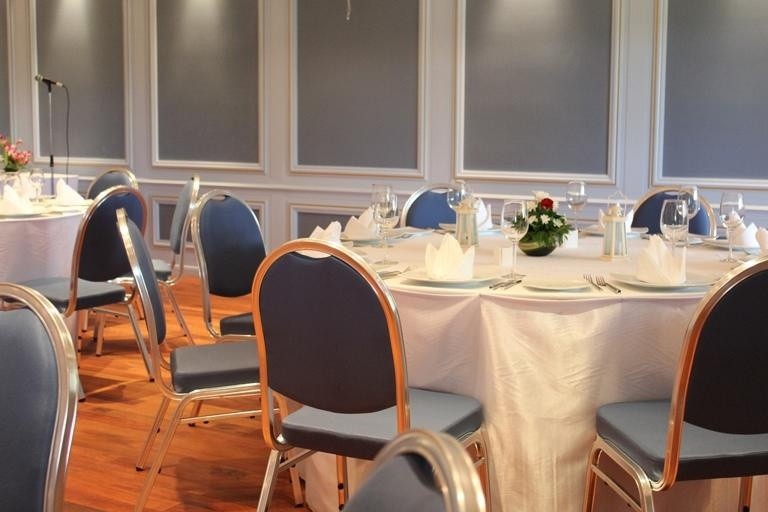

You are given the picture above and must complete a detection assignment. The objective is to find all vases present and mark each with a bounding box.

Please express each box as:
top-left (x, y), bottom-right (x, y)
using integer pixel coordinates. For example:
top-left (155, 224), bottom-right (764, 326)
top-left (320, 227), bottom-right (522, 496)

top-left (516, 223), bottom-right (558, 257)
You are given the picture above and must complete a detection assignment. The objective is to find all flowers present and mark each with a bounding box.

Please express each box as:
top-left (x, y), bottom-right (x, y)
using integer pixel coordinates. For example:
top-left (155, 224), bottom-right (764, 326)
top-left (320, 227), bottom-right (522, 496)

top-left (0, 133), bottom-right (32, 172)
top-left (511, 198), bottom-right (573, 247)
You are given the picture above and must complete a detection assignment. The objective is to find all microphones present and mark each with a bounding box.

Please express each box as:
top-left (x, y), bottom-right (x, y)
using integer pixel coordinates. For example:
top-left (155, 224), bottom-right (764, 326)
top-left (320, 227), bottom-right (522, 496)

top-left (34, 74), bottom-right (64, 87)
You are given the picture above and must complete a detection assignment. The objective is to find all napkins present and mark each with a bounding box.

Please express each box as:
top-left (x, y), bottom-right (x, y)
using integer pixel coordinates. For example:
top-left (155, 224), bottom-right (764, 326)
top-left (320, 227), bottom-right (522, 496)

top-left (726, 215), bottom-right (760, 247)
top-left (525, 260), bottom-right (586, 289)
top-left (346, 205), bottom-right (386, 239)
top-left (424, 232), bottom-right (475, 283)
top-left (632, 235), bottom-right (688, 284)
top-left (595, 208), bottom-right (634, 235)
top-left (308, 220), bottom-right (349, 250)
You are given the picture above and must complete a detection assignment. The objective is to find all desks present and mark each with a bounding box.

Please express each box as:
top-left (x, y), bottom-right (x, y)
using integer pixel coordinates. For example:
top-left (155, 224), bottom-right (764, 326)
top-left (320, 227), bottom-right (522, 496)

top-left (296, 228), bottom-right (764, 512)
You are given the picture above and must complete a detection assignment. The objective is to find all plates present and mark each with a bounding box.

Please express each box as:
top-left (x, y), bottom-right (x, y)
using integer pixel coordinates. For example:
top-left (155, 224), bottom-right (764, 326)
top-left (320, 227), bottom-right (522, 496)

top-left (306, 180), bottom-right (755, 292)
top-left (0, 210), bottom-right (46, 216)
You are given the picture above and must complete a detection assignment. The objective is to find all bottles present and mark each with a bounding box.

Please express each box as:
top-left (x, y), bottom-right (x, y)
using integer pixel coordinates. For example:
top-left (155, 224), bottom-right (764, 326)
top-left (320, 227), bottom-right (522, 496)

top-left (2, 169), bottom-right (46, 203)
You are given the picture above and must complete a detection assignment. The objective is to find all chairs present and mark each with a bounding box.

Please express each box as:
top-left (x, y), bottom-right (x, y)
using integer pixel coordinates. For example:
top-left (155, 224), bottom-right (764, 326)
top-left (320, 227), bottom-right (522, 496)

top-left (0, 168), bottom-right (200, 402)
top-left (252, 238), bottom-right (492, 511)
top-left (584, 258), bottom-right (764, 510)
top-left (400, 183), bottom-right (488, 226)
top-left (1, 283), bottom-right (79, 512)
top-left (187, 189), bottom-right (267, 429)
top-left (630, 187), bottom-right (712, 235)
top-left (116, 207), bottom-right (303, 512)
